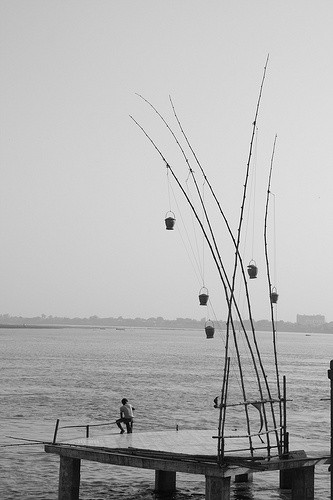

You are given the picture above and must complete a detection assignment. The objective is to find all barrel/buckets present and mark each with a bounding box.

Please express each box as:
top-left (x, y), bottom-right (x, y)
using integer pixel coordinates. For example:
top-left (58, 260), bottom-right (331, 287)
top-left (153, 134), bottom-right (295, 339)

top-left (247, 259), bottom-right (258, 278)
top-left (205, 320), bottom-right (214, 338)
top-left (270, 286), bottom-right (278, 304)
top-left (199, 287), bottom-right (209, 305)
top-left (165, 210), bottom-right (176, 231)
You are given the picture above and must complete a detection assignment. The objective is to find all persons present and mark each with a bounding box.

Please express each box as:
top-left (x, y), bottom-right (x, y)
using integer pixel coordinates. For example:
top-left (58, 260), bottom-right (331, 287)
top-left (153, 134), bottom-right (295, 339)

top-left (116, 398), bottom-right (135, 434)
